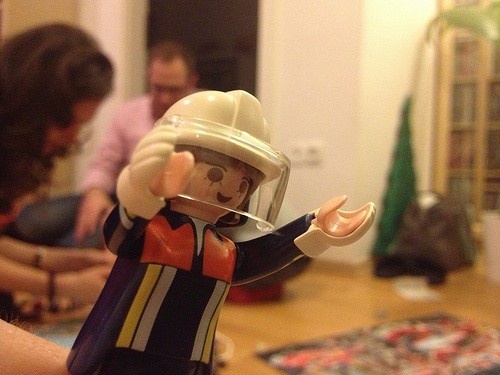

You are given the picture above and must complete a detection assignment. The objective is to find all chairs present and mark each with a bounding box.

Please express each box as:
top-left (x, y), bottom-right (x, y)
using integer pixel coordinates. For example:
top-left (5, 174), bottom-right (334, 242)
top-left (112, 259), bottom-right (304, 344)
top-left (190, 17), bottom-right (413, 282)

top-left (309, 256), bottom-right (356, 275)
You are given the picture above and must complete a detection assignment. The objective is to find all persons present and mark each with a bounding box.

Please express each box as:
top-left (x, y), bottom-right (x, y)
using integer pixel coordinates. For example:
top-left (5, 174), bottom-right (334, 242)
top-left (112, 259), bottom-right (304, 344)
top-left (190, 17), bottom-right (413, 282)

top-left (73, 41), bottom-right (210, 242)
top-left (0, 22), bottom-right (120, 307)
top-left (65, 88), bottom-right (375, 374)
top-left (0, 317), bottom-right (75, 375)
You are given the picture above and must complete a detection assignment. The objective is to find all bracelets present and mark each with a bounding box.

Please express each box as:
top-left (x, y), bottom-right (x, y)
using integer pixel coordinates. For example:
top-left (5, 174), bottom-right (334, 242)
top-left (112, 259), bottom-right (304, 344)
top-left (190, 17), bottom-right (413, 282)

top-left (33, 243), bottom-right (45, 271)
top-left (46, 269), bottom-right (57, 302)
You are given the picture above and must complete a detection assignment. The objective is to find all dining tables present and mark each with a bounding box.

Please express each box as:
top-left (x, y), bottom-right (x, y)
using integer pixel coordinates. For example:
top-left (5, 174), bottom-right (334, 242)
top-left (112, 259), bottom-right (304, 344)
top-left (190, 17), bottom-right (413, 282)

top-left (209, 272), bottom-right (500, 375)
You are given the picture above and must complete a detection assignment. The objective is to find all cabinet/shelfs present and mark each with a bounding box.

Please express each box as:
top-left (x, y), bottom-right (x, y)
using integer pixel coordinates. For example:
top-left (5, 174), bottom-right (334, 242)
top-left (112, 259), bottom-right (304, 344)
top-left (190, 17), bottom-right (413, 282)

top-left (431, 1), bottom-right (499, 214)
